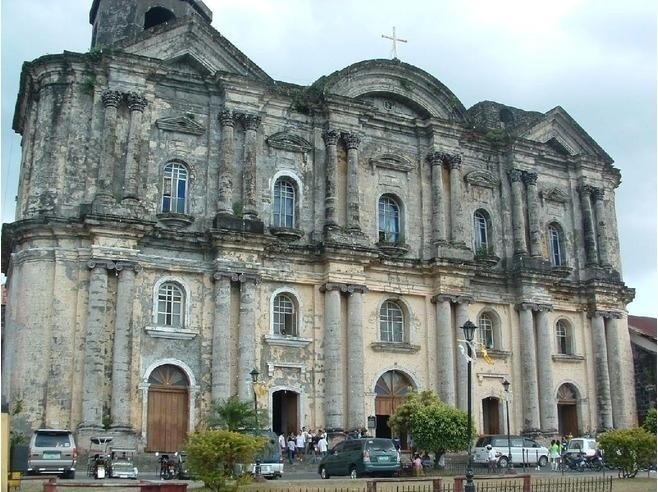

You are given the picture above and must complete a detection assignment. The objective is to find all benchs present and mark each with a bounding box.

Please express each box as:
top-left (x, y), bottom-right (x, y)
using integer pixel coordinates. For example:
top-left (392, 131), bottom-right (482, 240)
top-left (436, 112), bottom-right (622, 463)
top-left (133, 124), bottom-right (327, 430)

top-left (365, 473), bottom-right (532, 492)
top-left (8, 475), bottom-right (189, 492)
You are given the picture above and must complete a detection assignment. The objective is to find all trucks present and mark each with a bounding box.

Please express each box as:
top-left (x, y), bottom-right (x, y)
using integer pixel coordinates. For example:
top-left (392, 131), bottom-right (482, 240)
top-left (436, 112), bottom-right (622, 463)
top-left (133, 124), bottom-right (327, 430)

top-left (223, 426), bottom-right (286, 480)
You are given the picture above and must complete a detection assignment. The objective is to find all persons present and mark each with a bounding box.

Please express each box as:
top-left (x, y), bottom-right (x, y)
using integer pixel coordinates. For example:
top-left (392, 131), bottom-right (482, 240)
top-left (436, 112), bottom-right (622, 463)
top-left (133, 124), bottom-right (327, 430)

top-left (486, 444), bottom-right (500, 473)
top-left (392, 434), bottom-right (402, 463)
top-left (354, 427), bottom-right (364, 439)
top-left (548, 439), bottom-right (558, 471)
top-left (412, 452), bottom-right (425, 477)
top-left (421, 452), bottom-right (432, 470)
top-left (555, 440), bottom-right (564, 463)
top-left (278, 424), bottom-right (328, 467)
top-left (561, 436), bottom-right (568, 450)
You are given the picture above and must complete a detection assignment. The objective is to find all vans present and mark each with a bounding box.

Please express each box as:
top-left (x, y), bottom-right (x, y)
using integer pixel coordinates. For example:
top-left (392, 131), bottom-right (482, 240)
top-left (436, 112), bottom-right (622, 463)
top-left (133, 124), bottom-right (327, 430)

top-left (470, 431), bottom-right (552, 470)
top-left (317, 436), bottom-right (401, 481)
top-left (25, 429), bottom-right (78, 481)
top-left (565, 437), bottom-right (624, 466)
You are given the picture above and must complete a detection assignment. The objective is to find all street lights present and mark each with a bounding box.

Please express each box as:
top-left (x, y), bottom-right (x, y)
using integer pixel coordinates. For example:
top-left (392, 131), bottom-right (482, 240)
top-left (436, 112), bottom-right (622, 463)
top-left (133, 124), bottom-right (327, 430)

top-left (459, 319), bottom-right (479, 491)
top-left (501, 379), bottom-right (518, 476)
top-left (249, 368), bottom-right (262, 482)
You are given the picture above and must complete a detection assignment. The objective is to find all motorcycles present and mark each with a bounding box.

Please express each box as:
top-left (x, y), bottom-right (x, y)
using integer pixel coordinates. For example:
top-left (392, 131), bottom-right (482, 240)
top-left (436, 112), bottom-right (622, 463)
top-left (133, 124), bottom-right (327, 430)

top-left (87, 436), bottom-right (114, 480)
top-left (556, 449), bottom-right (656, 479)
top-left (400, 456), bottom-right (434, 474)
top-left (153, 451), bottom-right (179, 480)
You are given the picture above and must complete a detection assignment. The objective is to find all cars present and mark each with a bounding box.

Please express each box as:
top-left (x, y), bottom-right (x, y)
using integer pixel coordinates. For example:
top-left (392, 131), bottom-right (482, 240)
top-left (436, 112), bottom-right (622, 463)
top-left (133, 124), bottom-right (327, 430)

top-left (178, 453), bottom-right (198, 480)
